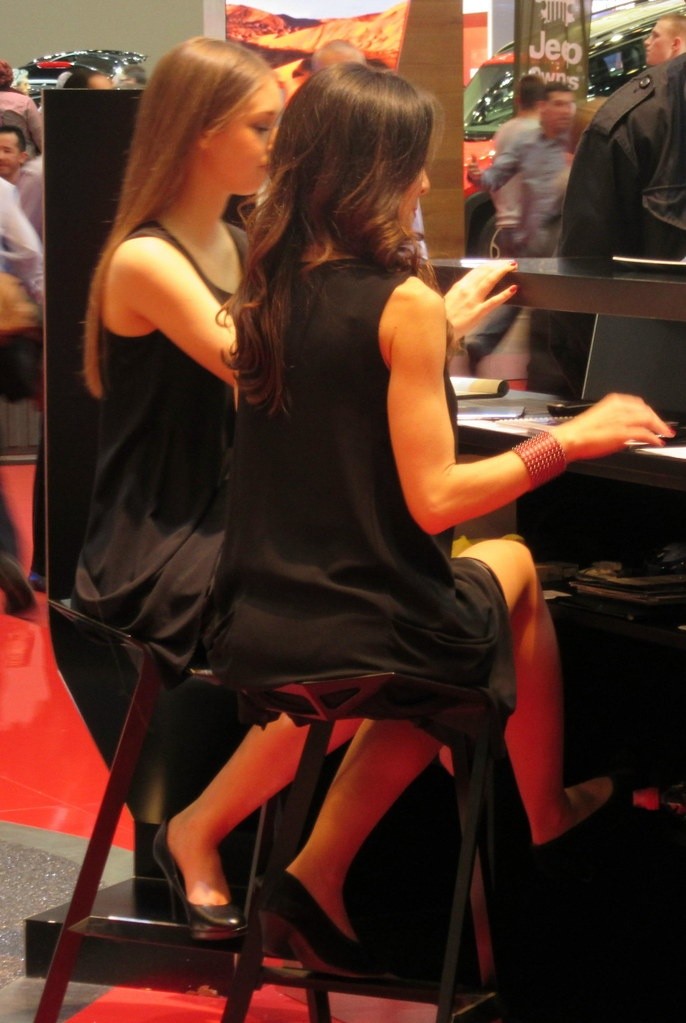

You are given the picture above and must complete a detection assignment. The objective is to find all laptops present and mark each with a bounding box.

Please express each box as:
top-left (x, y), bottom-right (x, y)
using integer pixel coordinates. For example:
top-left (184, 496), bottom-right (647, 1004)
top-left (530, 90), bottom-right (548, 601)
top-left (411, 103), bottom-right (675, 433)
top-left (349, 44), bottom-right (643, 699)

top-left (453, 314), bottom-right (686, 443)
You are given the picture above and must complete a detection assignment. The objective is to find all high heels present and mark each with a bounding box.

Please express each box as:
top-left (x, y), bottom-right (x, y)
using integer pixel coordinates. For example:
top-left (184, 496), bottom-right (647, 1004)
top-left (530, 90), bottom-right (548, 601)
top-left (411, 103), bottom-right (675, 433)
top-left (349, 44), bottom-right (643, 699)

top-left (526, 764), bottom-right (633, 871)
top-left (252, 869), bottom-right (400, 980)
top-left (150, 813), bottom-right (249, 940)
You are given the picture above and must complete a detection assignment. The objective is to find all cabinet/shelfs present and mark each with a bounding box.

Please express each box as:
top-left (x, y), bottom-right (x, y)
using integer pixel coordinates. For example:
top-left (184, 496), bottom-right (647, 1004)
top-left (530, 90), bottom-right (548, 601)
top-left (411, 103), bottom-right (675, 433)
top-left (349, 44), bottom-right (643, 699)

top-left (429, 257), bottom-right (686, 652)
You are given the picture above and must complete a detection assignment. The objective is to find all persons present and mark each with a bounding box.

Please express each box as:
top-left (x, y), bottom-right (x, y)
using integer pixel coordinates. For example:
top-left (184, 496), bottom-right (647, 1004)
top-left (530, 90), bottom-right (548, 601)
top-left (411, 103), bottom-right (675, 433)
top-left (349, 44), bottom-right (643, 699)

top-left (311, 41), bottom-right (429, 261)
top-left (70, 35), bottom-right (366, 933)
top-left (203, 63), bottom-right (674, 984)
top-left (464, 11), bottom-right (686, 403)
top-left (1, 62), bottom-right (151, 611)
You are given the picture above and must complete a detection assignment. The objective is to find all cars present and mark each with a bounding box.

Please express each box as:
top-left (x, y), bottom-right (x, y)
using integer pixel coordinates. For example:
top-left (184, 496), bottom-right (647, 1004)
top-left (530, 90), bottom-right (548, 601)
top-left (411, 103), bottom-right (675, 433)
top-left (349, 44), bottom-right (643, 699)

top-left (13, 49), bottom-right (149, 108)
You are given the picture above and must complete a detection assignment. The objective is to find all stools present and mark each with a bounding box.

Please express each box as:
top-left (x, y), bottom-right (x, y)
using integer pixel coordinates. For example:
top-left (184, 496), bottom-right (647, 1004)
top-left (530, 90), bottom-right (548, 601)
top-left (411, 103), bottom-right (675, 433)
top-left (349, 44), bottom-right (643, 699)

top-left (223, 666), bottom-right (493, 1023)
top-left (33, 598), bottom-right (162, 1023)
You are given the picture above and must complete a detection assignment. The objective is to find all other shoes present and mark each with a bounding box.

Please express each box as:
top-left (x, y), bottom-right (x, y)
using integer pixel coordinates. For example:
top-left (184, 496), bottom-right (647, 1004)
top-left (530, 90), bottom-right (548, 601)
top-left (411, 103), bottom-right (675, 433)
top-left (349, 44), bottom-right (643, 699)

top-left (0, 555), bottom-right (37, 611)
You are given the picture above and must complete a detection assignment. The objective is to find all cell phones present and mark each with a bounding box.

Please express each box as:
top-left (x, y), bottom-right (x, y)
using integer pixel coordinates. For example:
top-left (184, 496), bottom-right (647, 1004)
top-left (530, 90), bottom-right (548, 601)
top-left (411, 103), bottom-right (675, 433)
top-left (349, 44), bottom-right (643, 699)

top-left (456, 406), bottom-right (527, 419)
top-left (546, 398), bottom-right (595, 418)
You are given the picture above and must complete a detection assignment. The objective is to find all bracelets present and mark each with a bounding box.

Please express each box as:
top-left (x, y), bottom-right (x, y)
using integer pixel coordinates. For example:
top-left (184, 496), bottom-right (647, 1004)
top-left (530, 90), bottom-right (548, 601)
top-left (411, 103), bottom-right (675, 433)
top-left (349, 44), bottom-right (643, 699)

top-left (511, 430), bottom-right (567, 492)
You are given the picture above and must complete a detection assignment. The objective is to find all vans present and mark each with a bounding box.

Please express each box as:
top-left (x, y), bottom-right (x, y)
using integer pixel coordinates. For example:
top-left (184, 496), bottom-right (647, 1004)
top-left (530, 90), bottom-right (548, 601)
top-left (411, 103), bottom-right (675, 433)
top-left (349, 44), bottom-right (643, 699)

top-left (464, 0), bottom-right (686, 262)
